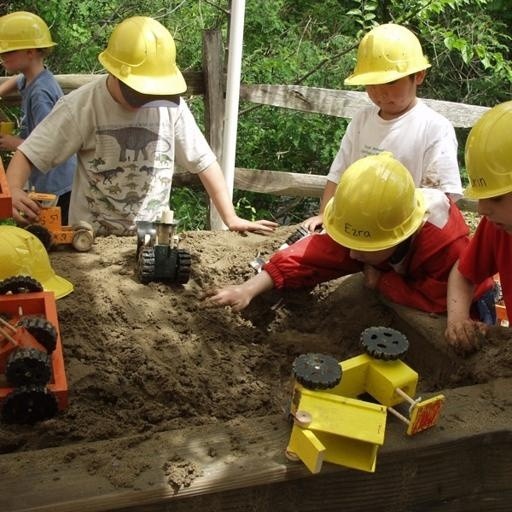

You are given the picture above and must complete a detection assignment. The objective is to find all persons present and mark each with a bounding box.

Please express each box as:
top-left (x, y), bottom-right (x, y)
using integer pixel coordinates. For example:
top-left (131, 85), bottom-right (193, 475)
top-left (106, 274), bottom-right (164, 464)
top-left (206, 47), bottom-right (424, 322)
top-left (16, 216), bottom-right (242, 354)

top-left (298, 22), bottom-right (466, 233)
top-left (441, 98), bottom-right (511, 357)
top-left (207, 150), bottom-right (501, 329)
top-left (0, 11), bottom-right (77, 228)
top-left (4, 13), bottom-right (279, 236)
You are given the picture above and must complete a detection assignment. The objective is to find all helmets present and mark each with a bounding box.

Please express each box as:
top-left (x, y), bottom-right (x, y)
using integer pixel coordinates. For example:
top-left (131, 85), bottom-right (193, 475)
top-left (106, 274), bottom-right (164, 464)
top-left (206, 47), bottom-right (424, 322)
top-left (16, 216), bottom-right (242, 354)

top-left (459, 98), bottom-right (512, 201)
top-left (98, 15), bottom-right (188, 97)
top-left (321, 154), bottom-right (426, 254)
top-left (341, 22), bottom-right (436, 88)
top-left (0, 223), bottom-right (73, 301)
top-left (0, 11), bottom-right (59, 54)
